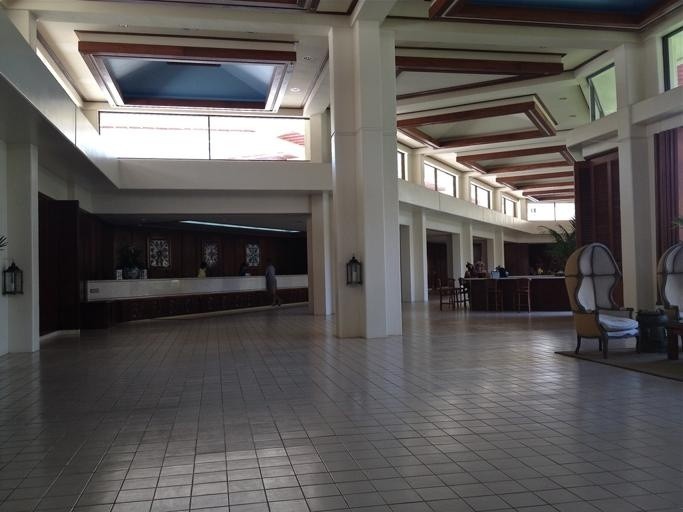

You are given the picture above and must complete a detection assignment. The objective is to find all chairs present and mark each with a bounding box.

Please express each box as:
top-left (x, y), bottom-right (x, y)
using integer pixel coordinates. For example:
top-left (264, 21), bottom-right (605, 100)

top-left (3, 262), bottom-right (24, 296)
top-left (436, 277), bottom-right (468, 311)
top-left (563, 240), bottom-right (683, 359)
top-left (510, 277), bottom-right (533, 313)
top-left (485, 276), bottom-right (505, 311)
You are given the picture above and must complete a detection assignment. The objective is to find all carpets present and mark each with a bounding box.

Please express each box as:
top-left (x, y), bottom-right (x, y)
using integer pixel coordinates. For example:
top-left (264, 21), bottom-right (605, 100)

top-left (554, 347), bottom-right (683, 381)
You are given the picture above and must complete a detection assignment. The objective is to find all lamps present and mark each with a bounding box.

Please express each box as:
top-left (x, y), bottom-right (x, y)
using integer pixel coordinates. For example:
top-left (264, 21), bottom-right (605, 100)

top-left (346, 256), bottom-right (362, 284)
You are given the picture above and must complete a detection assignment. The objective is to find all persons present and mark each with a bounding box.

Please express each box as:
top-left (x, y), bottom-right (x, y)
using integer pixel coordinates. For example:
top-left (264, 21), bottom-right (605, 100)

top-left (462, 262), bottom-right (486, 304)
top-left (239, 262), bottom-right (252, 276)
top-left (197, 261), bottom-right (208, 278)
top-left (264, 257), bottom-right (283, 307)
